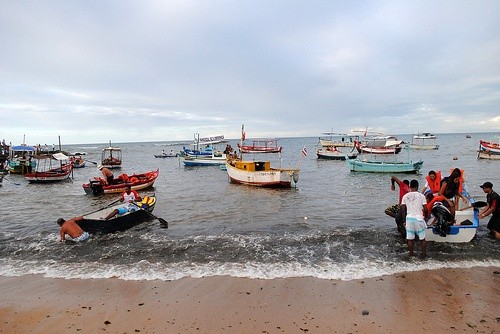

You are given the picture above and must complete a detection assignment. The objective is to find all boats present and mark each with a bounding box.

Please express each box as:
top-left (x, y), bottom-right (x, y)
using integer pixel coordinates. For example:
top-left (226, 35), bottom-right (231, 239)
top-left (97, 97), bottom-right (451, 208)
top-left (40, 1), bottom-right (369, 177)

top-left (237, 139), bottom-right (282, 153)
top-left (226, 158), bottom-right (301, 187)
top-left (477, 140), bottom-right (500, 160)
top-left (397, 178), bottom-right (481, 244)
top-left (82, 168), bottom-right (159, 196)
top-left (154, 133), bottom-right (240, 166)
top-left (316, 125), bottom-right (408, 160)
top-left (0, 135), bottom-right (86, 186)
top-left (345, 154), bottom-right (423, 174)
top-left (414, 132), bottom-right (436, 139)
top-left (403, 137), bottom-right (440, 150)
top-left (98, 140), bottom-right (123, 169)
top-left (74, 192), bottom-right (157, 235)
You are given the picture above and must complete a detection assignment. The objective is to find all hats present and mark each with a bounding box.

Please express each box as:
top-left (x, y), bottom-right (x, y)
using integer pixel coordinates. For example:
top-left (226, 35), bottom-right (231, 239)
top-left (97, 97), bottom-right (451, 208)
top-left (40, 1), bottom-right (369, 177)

top-left (480, 182), bottom-right (493, 189)
top-left (97, 165), bottom-right (103, 170)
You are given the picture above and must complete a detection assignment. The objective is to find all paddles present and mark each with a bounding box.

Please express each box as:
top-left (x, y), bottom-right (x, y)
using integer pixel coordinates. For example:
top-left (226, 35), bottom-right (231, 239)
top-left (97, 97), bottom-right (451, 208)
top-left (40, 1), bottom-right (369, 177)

top-left (132, 202), bottom-right (167, 225)
top-left (2, 177), bottom-right (20, 185)
top-left (458, 201), bottom-right (487, 211)
top-left (83, 199), bottom-right (119, 216)
top-left (82, 159), bottom-right (97, 164)
top-left (456, 219), bottom-right (473, 225)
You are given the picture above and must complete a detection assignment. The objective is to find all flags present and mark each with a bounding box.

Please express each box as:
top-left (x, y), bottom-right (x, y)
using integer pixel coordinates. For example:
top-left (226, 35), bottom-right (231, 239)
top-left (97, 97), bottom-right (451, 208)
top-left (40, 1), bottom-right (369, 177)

top-left (364, 126), bottom-right (367, 137)
top-left (302, 147), bottom-right (308, 157)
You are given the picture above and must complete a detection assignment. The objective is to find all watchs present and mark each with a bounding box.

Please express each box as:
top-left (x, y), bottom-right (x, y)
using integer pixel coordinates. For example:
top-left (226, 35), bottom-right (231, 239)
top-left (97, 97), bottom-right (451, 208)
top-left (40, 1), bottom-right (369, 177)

top-left (482, 214), bottom-right (485, 217)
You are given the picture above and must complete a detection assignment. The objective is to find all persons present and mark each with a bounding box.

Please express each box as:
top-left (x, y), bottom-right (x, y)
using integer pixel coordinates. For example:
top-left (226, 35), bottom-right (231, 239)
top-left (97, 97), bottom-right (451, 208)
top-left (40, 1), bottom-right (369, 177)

top-left (225, 143), bottom-right (239, 164)
top-left (97, 163), bottom-right (114, 184)
top-left (390, 168), bottom-right (500, 258)
top-left (99, 184), bottom-right (142, 220)
top-left (56, 216), bottom-right (90, 242)
top-left (162, 149), bottom-right (174, 155)
top-left (323, 145), bottom-right (337, 153)
top-left (0, 140), bottom-right (83, 175)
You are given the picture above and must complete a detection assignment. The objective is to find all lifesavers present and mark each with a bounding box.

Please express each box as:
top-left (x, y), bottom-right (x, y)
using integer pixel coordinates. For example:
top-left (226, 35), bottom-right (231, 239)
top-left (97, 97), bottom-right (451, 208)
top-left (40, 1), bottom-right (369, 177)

top-left (130, 177), bottom-right (138, 182)
